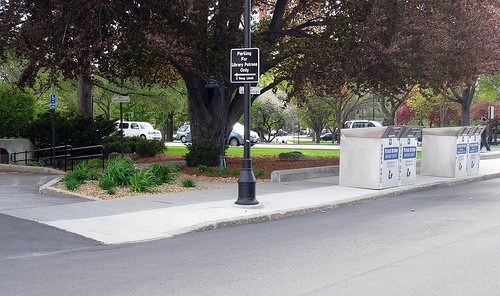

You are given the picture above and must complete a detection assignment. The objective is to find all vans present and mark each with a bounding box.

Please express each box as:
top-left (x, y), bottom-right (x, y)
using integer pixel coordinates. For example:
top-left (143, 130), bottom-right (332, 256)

top-left (344, 120), bottom-right (383, 128)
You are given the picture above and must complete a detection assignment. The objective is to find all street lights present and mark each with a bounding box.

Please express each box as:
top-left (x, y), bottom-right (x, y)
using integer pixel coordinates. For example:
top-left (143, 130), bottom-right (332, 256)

top-left (367, 89), bottom-right (374, 120)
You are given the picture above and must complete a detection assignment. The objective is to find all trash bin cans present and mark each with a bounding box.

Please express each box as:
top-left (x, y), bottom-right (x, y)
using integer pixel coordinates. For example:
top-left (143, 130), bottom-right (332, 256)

top-left (421, 125), bottom-right (487, 178)
top-left (339, 124), bottom-right (422, 192)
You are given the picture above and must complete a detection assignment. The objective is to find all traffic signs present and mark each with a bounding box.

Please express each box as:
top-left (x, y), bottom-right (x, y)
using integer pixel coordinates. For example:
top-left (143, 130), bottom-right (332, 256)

top-left (229, 48), bottom-right (260, 84)
top-left (111, 95), bottom-right (131, 103)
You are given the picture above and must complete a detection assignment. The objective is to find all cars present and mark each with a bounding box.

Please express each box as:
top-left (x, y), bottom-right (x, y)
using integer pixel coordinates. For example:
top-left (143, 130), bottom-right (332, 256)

top-left (270, 129), bottom-right (288, 136)
top-left (175, 124), bottom-right (191, 143)
top-left (102, 120), bottom-right (162, 142)
top-left (320, 128), bottom-right (339, 141)
top-left (227, 122), bottom-right (260, 147)
top-left (300, 129), bottom-right (309, 135)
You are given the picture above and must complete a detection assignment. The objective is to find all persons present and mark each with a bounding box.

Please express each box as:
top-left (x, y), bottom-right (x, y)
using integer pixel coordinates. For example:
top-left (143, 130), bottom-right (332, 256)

top-left (479, 116), bottom-right (492, 152)
